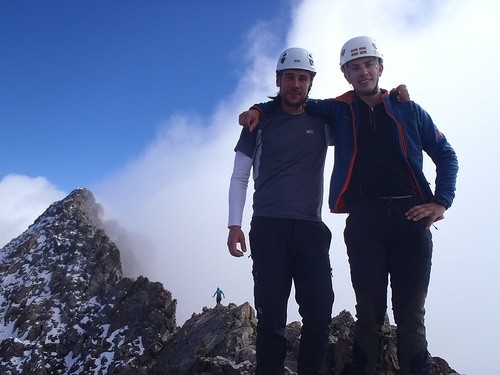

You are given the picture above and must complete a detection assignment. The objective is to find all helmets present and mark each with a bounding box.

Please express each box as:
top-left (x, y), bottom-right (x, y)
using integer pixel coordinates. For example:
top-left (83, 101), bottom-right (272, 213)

top-left (276, 47), bottom-right (316, 75)
top-left (339, 35), bottom-right (384, 68)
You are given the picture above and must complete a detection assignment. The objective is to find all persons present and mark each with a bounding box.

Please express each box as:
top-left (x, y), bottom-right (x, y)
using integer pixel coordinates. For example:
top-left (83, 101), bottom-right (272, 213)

top-left (212, 287), bottom-right (225, 304)
top-left (227, 48), bottom-right (412, 375)
top-left (238, 34), bottom-right (460, 374)
